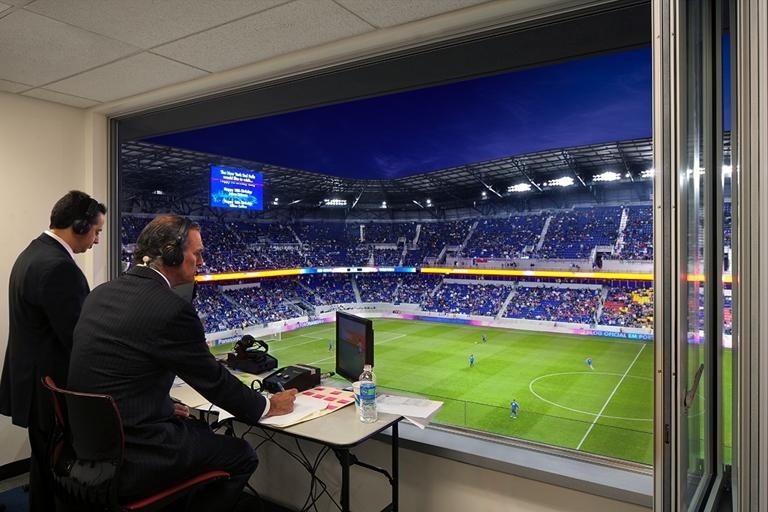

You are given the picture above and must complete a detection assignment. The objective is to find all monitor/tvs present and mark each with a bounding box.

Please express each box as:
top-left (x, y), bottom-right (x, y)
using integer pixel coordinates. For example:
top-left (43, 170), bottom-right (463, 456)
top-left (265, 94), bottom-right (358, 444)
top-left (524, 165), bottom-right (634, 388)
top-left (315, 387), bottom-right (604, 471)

top-left (336, 311), bottom-right (374, 391)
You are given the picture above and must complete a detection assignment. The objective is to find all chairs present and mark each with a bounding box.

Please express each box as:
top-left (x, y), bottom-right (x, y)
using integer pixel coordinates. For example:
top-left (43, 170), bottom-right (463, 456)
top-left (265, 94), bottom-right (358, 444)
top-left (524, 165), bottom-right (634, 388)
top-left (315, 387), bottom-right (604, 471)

top-left (41, 376), bottom-right (230, 511)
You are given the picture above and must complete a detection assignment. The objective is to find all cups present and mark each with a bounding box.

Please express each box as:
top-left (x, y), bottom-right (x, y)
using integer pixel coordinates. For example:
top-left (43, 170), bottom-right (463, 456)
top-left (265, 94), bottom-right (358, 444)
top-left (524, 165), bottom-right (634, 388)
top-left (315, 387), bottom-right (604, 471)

top-left (352, 380), bottom-right (360, 414)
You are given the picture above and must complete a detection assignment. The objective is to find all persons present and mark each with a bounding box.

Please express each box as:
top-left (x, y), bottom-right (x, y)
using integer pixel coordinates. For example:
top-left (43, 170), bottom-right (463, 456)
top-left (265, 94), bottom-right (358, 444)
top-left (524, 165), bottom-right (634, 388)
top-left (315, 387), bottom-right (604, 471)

top-left (0, 190), bottom-right (108, 512)
top-left (469, 354), bottom-right (474, 367)
top-left (116, 203), bottom-right (733, 341)
top-left (586, 354), bottom-right (595, 370)
top-left (65, 214), bottom-right (298, 512)
top-left (510, 399), bottom-right (519, 419)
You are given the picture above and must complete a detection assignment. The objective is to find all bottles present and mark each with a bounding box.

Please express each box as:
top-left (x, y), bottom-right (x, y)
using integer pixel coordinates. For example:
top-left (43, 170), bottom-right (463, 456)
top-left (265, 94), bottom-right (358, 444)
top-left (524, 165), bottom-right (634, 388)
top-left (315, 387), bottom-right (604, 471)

top-left (360, 365), bottom-right (378, 423)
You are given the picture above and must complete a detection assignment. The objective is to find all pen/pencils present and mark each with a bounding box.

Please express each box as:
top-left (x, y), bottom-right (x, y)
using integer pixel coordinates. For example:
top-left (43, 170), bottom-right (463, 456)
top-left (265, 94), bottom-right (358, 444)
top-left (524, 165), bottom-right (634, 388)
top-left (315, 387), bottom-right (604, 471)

top-left (277, 381), bottom-right (285, 392)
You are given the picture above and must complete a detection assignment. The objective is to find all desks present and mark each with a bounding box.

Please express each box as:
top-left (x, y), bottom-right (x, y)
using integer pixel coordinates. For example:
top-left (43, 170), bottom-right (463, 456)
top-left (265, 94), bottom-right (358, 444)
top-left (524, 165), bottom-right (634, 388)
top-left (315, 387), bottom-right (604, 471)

top-left (168, 365), bottom-right (405, 511)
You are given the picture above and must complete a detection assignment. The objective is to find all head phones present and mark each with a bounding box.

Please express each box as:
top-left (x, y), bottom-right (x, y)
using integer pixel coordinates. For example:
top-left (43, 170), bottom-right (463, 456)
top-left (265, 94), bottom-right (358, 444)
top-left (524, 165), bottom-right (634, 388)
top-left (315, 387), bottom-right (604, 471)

top-left (233, 334), bottom-right (269, 359)
top-left (162, 217), bottom-right (191, 266)
top-left (71, 199), bottom-right (99, 234)
